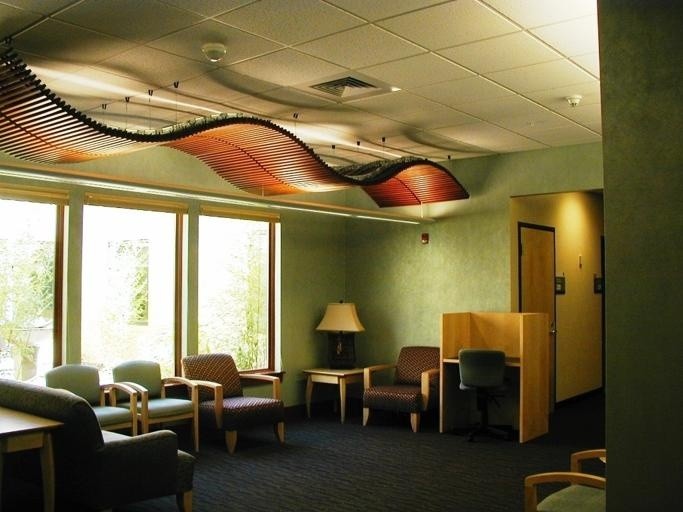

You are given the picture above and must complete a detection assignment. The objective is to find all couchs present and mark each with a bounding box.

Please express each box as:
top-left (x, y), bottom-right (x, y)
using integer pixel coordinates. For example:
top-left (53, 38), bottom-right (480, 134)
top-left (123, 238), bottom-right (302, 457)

top-left (1, 379), bottom-right (196, 512)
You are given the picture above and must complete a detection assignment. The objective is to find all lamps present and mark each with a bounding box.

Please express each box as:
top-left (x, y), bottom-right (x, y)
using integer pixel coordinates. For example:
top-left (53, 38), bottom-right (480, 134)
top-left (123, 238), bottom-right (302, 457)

top-left (312, 298), bottom-right (368, 368)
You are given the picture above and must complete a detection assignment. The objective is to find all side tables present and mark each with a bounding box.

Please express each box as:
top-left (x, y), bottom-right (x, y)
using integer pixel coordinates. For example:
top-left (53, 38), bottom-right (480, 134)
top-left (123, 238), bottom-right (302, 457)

top-left (300, 365), bottom-right (363, 426)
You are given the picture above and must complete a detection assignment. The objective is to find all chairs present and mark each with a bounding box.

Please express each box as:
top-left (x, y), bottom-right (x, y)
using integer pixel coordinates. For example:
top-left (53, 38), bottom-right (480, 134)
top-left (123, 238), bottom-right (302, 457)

top-left (109, 360), bottom-right (199, 453)
top-left (45, 364), bottom-right (137, 437)
top-left (182, 353), bottom-right (285, 455)
top-left (361, 343), bottom-right (514, 444)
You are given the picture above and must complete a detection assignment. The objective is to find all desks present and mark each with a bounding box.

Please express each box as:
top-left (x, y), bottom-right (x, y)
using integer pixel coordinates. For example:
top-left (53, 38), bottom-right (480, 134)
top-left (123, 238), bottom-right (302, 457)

top-left (438, 310), bottom-right (550, 443)
top-left (1, 407), bottom-right (65, 511)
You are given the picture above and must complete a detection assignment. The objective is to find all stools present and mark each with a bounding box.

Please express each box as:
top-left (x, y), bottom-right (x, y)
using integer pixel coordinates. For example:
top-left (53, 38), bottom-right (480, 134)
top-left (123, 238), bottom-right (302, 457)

top-left (522, 448), bottom-right (606, 511)
